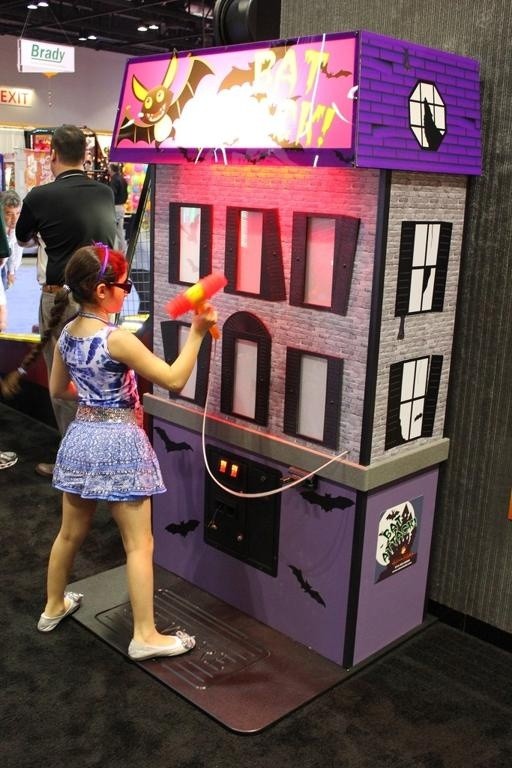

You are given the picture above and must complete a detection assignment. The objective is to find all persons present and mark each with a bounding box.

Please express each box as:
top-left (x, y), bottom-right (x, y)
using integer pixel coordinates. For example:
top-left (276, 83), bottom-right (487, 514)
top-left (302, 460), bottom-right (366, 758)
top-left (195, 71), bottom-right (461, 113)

top-left (0, 240), bottom-right (223, 664)
top-left (16, 124), bottom-right (121, 481)
top-left (0, 207), bottom-right (20, 472)
top-left (107, 158), bottom-right (128, 231)
top-left (0, 187), bottom-right (22, 288)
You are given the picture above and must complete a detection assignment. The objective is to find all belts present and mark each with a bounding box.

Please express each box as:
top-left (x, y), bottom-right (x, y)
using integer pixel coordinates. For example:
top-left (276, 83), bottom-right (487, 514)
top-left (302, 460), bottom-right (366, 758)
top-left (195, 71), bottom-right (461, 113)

top-left (39, 283), bottom-right (63, 293)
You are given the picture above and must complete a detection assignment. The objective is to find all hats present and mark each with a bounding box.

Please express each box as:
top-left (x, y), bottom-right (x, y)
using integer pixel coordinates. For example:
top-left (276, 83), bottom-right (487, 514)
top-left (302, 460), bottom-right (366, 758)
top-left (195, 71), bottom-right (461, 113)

top-left (93, 280), bottom-right (133, 294)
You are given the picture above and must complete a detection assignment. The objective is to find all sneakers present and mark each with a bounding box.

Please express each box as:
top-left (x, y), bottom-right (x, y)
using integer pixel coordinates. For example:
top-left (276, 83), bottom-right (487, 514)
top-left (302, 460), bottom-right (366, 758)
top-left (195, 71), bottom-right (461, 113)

top-left (35, 463), bottom-right (55, 476)
top-left (128, 631), bottom-right (195, 661)
top-left (37, 592), bottom-right (84, 633)
top-left (0, 450), bottom-right (19, 470)
